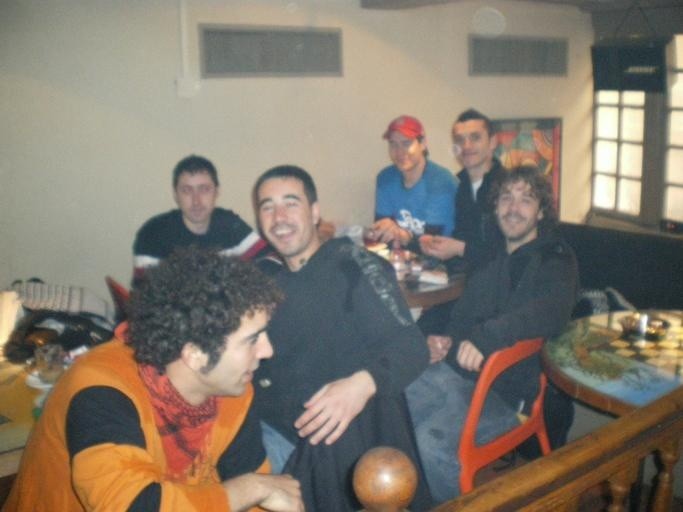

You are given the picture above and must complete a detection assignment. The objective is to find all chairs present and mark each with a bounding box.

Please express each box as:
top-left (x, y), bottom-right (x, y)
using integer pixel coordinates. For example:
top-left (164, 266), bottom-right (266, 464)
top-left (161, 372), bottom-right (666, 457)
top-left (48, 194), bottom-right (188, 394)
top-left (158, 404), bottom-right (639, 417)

top-left (458, 340), bottom-right (551, 494)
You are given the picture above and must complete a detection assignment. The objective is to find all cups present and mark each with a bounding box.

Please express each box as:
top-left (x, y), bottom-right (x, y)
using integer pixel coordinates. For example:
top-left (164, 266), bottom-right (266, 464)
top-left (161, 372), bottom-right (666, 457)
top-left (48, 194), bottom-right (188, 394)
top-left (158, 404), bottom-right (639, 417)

top-left (23, 343), bottom-right (65, 384)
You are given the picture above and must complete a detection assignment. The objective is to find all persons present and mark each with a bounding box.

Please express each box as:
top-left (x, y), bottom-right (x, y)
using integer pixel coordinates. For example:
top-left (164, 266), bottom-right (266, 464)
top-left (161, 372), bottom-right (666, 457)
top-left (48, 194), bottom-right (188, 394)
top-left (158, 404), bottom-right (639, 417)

top-left (402, 166), bottom-right (581, 502)
top-left (418, 107), bottom-right (513, 268)
top-left (132, 153), bottom-right (284, 295)
top-left (252, 164), bottom-right (431, 511)
top-left (1, 241), bottom-right (304, 512)
top-left (369, 114), bottom-right (461, 251)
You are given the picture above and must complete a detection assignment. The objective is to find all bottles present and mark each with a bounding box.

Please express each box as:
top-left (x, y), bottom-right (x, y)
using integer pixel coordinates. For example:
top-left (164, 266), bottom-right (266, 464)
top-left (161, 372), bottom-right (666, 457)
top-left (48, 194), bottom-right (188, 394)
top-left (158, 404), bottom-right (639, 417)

top-left (388, 232), bottom-right (405, 284)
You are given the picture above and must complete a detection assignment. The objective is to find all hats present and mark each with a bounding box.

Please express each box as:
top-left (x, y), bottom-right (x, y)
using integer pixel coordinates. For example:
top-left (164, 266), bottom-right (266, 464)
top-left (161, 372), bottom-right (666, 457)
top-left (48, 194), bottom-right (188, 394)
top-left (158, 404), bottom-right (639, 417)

top-left (383, 116), bottom-right (422, 138)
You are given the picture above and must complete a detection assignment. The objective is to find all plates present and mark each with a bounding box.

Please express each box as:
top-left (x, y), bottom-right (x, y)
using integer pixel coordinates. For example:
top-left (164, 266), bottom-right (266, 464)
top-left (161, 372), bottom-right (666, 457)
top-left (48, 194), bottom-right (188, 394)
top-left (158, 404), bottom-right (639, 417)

top-left (24, 354), bottom-right (69, 390)
